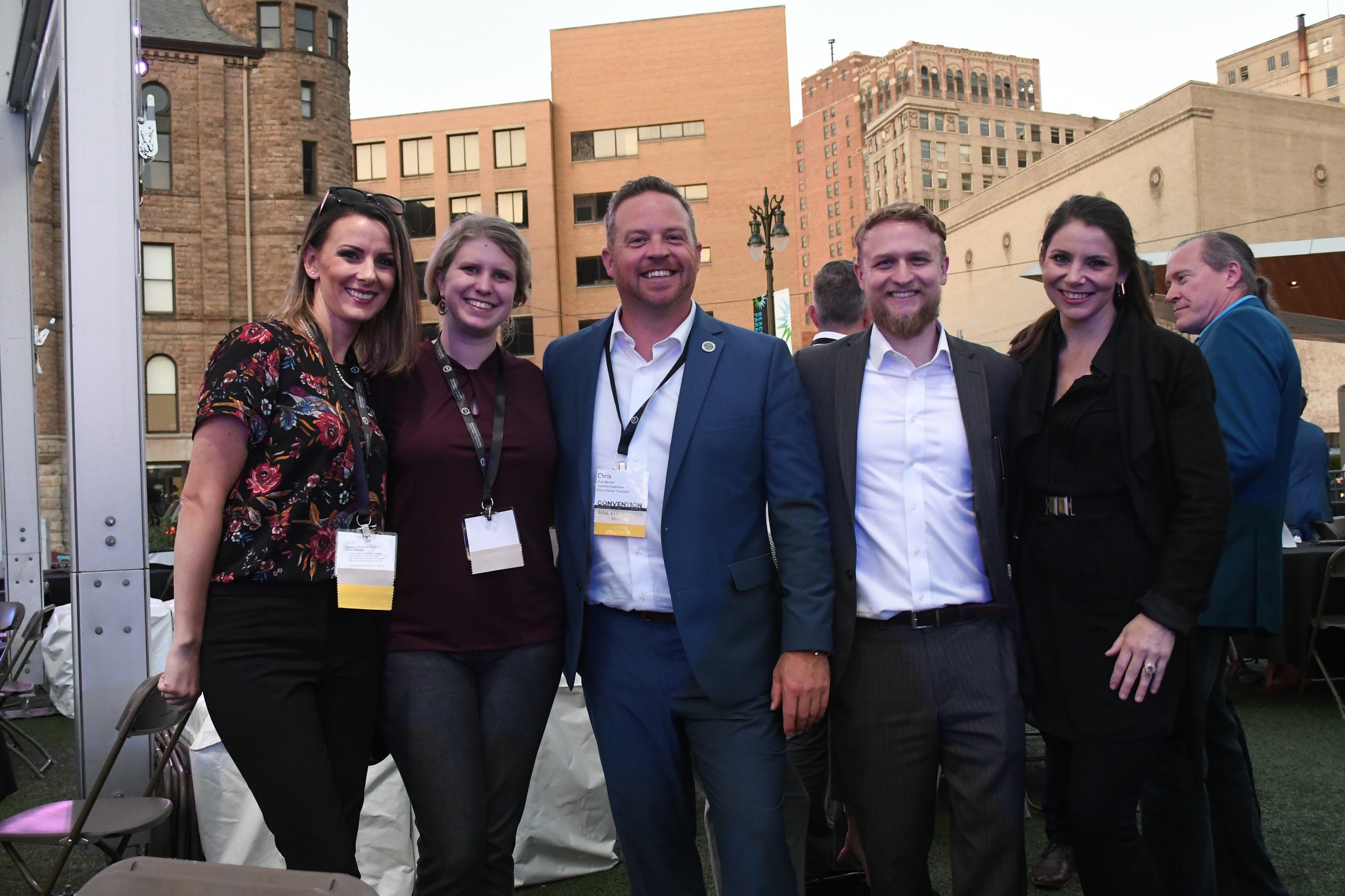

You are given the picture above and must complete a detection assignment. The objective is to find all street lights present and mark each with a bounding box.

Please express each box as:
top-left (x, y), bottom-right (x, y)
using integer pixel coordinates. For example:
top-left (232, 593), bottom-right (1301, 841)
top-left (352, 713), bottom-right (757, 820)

top-left (747, 186), bottom-right (790, 337)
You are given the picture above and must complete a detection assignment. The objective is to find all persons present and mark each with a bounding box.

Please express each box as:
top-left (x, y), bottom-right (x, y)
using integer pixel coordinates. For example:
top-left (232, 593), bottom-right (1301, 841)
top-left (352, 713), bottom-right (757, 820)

top-left (155, 190), bottom-right (429, 881)
top-left (542, 171), bottom-right (834, 894)
top-left (1282, 386), bottom-right (1334, 543)
top-left (1138, 233), bottom-right (1302, 895)
top-left (808, 261), bottom-right (873, 347)
top-left (358, 216), bottom-right (574, 896)
top-left (1010, 197), bottom-right (1227, 896)
top-left (788, 200), bottom-right (1020, 896)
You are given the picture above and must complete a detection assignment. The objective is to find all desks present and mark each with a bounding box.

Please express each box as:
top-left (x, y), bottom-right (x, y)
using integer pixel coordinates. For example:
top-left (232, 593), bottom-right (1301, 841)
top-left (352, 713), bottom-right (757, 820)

top-left (1239, 541), bottom-right (1345, 658)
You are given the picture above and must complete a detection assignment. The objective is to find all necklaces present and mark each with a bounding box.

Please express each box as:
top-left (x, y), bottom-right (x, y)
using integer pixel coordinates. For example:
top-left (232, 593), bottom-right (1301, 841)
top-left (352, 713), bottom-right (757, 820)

top-left (298, 316), bottom-right (357, 391)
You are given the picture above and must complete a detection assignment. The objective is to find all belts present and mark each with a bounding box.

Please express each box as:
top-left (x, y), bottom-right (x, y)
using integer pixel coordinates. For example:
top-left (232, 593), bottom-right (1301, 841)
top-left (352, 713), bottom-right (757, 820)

top-left (859, 607), bottom-right (994, 628)
top-left (1030, 491), bottom-right (1137, 521)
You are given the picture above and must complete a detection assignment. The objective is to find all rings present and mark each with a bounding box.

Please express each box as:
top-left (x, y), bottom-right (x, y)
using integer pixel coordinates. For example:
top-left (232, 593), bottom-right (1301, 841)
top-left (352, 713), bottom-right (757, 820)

top-left (1142, 663), bottom-right (1156, 674)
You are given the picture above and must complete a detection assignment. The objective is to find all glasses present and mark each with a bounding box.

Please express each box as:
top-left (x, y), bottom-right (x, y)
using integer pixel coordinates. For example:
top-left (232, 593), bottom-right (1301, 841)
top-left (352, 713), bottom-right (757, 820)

top-left (317, 186), bottom-right (410, 223)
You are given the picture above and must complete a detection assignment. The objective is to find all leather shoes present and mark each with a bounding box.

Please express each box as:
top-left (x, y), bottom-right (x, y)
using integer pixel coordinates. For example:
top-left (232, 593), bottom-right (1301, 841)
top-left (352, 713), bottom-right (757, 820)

top-left (1029, 842), bottom-right (1073, 889)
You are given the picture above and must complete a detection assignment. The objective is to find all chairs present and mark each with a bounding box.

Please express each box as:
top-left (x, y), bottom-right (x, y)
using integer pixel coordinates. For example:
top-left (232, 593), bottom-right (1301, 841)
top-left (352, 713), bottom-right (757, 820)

top-left (0, 602), bottom-right (25, 667)
top-left (1297, 546), bottom-right (1345, 721)
top-left (145, 730), bottom-right (198, 861)
top-left (0, 674), bottom-right (199, 896)
top-left (0, 604), bottom-right (58, 780)
